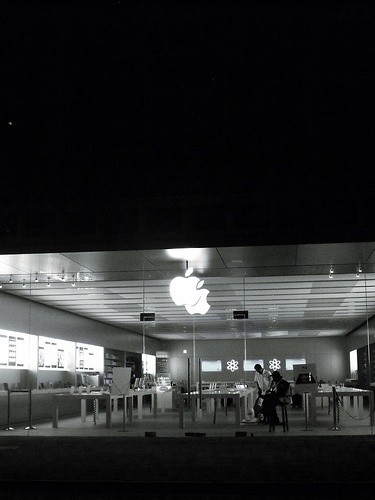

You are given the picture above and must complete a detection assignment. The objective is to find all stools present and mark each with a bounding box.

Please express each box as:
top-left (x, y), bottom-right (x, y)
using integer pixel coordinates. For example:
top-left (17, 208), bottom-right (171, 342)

top-left (269, 402), bottom-right (289, 432)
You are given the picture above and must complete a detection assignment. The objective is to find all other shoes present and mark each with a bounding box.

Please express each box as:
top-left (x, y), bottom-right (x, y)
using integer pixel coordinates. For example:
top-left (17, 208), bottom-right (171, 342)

top-left (291, 402), bottom-right (303, 408)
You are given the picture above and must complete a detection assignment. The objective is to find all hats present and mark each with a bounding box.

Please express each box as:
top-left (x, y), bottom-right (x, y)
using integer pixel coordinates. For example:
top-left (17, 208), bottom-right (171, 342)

top-left (270, 371), bottom-right (283, 377)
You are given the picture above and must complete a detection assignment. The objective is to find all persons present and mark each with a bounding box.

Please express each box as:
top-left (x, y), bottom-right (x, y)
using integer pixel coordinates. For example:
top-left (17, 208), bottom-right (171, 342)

top-left (254, 364), bottom-right (274, 423)
top-left (263, 372), bottom-right (290, 425)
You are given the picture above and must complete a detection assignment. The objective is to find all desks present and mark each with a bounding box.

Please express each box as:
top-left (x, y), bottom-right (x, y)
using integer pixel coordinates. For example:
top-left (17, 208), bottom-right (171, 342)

top-left (178, 386), bottom-right (257, 429)
top-left (304, 385), bottom-right (375, 427)
top-left (52, 389), bottom-right (157, 430)
